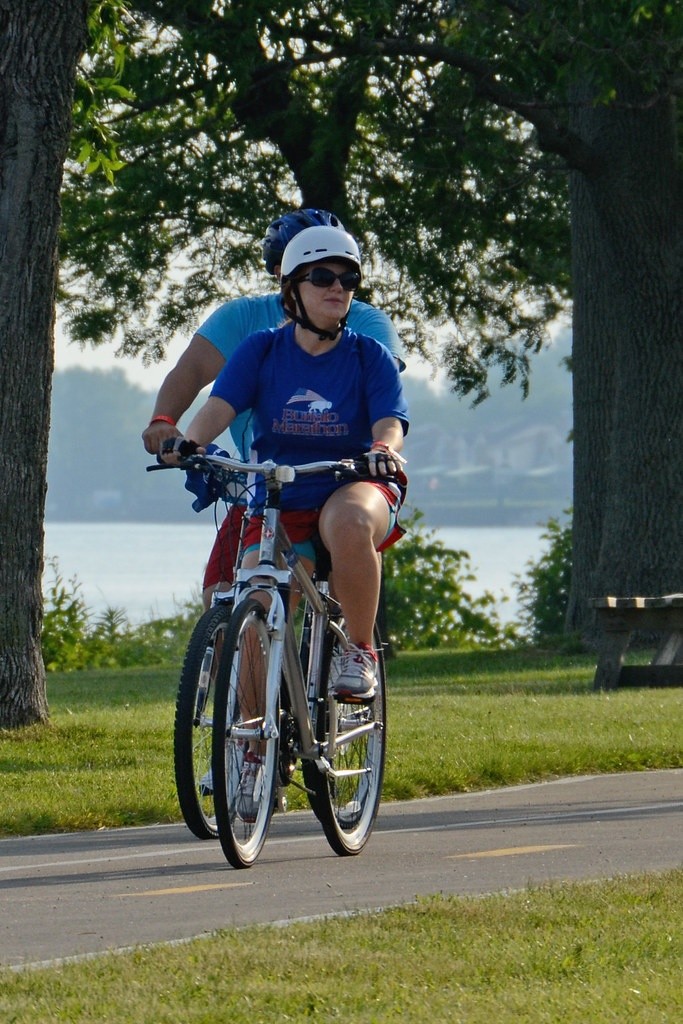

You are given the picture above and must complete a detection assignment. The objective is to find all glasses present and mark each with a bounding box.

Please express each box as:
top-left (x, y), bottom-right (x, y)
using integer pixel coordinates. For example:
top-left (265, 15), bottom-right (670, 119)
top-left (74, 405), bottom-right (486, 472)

top-left (297, 267), bottom-right (361, 292)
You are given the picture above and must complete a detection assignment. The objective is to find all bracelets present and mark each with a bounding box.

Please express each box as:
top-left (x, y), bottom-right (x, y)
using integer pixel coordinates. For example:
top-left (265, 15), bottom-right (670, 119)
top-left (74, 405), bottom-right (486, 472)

top-left (149, 416), bottom-right (175, 427)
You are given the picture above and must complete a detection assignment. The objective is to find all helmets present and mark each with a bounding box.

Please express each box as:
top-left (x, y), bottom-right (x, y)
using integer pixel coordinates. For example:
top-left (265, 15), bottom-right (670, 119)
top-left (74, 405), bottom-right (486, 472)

top-left (280, 226), bottom-right (361, 276)
top-left (262, 208), bottom-right (345, 276)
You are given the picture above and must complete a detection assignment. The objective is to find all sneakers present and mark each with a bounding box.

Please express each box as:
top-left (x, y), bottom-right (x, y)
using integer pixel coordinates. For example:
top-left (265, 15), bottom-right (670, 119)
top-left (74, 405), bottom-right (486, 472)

top-left (235, 752), bottom-right (266, 824)
top-left (199, 769), bottom-right (214, 797)
top-left (333, 642), bottom-right (379, 699)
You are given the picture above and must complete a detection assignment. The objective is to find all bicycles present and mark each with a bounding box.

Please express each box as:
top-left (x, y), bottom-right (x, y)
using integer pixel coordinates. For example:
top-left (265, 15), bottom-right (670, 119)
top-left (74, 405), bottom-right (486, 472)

top-left (147, 436), bottom-right (389, 867)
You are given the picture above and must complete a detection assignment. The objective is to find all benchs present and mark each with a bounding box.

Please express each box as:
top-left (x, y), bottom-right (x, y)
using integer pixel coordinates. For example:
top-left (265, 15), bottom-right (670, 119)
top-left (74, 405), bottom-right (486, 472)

top-left (588, 592), bottom-right (683, 690)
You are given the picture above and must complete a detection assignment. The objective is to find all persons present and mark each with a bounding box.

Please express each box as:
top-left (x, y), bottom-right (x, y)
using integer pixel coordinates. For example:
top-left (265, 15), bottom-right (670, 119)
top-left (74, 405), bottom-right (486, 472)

top-left (141, 210), bottom-right (406, 798)
top-left (156, 227), bottom-right (411, 822)
